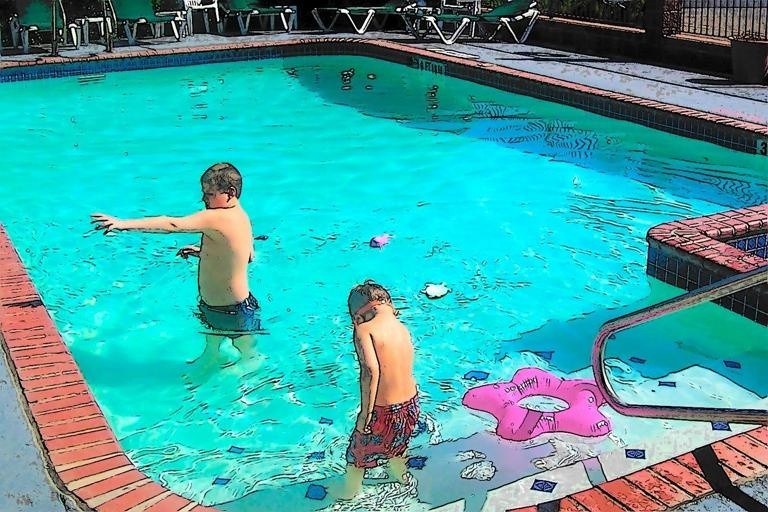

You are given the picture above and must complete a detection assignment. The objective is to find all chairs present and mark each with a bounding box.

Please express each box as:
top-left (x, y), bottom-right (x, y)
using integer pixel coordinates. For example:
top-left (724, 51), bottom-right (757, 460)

top-left (7, 1), bottom-right (543, 57)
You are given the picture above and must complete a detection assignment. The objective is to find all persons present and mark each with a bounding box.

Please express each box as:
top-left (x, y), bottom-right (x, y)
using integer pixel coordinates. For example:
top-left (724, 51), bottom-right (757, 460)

top-left (344, 280), bottom-right (420, 501)
top-left (90, 163), bottom-right (260, 361)
